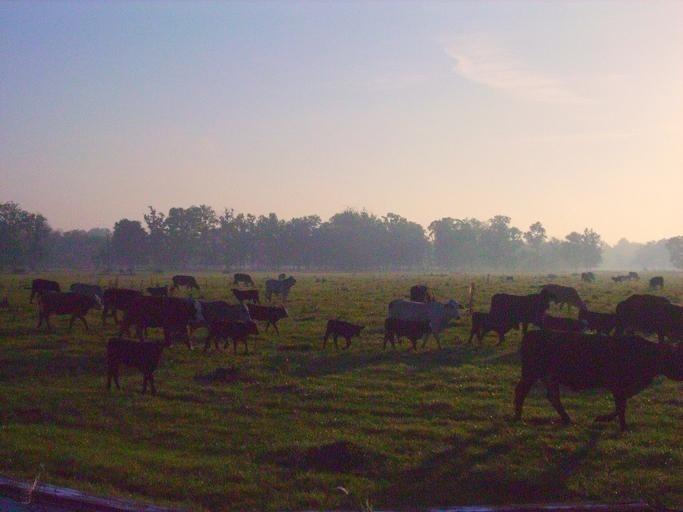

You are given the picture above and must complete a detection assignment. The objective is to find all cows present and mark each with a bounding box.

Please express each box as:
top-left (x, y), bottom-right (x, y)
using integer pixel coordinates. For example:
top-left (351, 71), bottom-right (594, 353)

top-left (468, 273), bottom-right (683, 346)
top-left (512, 330), bottom-right (682, 431)
top-left (384, 285), bottom-right (463, 350)
top-left (323, 320), bottom-right (365, 350)
top-left (29, 274), bottom-right (295, 353)
top-left (103, 338), bottom-right (166, 397)
